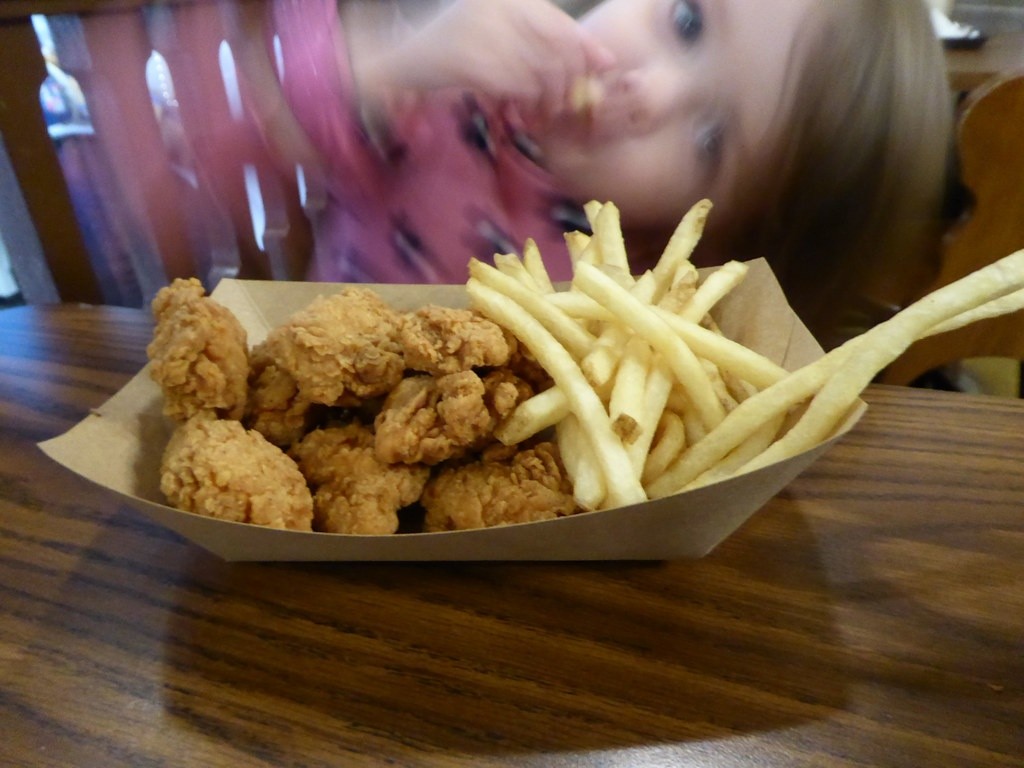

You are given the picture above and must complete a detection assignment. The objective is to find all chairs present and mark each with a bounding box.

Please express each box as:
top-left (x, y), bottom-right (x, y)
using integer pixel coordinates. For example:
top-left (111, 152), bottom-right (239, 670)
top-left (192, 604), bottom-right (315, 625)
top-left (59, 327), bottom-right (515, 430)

top-left (885, 68), bottom-right (1024, 399)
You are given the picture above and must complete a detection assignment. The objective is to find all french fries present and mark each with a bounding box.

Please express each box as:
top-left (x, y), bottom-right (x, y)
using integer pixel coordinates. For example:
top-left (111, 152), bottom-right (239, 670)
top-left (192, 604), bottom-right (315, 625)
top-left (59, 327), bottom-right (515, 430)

top-left (464, 199), bottom-right (1024, 507)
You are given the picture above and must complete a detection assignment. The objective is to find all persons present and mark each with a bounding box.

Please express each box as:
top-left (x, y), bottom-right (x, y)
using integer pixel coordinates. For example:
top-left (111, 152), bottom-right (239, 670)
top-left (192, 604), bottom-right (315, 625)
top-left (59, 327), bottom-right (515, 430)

top-left (39, 0), bottom-right (954, 326)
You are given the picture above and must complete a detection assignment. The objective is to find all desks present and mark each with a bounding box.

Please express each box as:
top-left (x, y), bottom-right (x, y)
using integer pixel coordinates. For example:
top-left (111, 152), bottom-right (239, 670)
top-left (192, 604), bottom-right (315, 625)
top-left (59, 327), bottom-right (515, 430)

top-left (0, 294), bottom-right (1024, 768)
top-left (916, 0), bottom-right (1023, 106)
